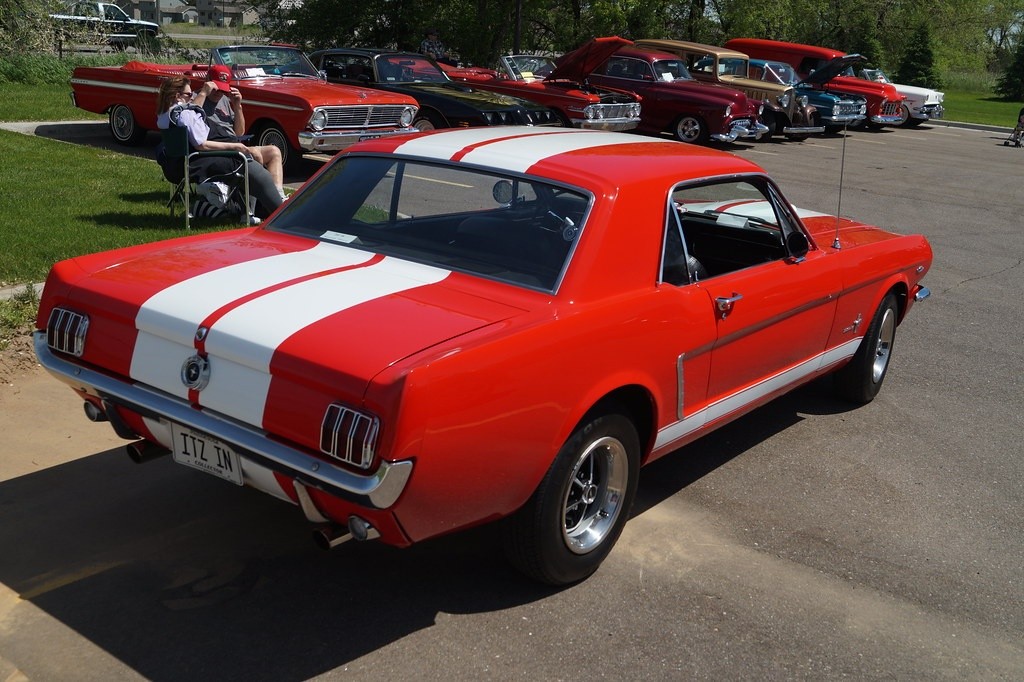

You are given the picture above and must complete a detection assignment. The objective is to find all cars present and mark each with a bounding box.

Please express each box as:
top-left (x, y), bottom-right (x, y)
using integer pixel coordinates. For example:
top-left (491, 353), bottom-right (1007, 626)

top-left (67, 43), bottom-right (424, 177)
top-left (30, 124), bottom-right (936, 589)
top-left (378, 36), bottom-right (644, 133)
top-left (533, 34), bottom-right (947, 235)
top-left (46, 0), bottom-right (160, 51)
top-left (275, 47), bottom-right (570, 131)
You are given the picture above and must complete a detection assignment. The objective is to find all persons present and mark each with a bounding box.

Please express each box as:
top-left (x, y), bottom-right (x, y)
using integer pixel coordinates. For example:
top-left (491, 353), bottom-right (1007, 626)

top-left (157, 76), bottom-right (283, 224)
top-left (1013, 115), bottom-right (1024, 140)
top-left (192, 64), bottom-right (287, 202)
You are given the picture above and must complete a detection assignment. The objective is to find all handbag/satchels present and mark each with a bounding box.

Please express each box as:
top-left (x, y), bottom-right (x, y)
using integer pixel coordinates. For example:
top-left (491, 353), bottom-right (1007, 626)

top-left (192, 182), bottom-right (231, 219)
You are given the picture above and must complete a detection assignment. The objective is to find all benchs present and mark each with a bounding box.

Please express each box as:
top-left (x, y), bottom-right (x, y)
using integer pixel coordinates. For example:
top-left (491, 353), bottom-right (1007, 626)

top-left (147, 65), bottom-right (191, 75)
top-left (450, 72), bottom-right (492, 81)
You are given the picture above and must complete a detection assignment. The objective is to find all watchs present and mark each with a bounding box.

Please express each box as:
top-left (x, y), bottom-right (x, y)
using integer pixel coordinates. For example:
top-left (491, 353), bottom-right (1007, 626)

top-left (234, 104), bottom-right (244, 109)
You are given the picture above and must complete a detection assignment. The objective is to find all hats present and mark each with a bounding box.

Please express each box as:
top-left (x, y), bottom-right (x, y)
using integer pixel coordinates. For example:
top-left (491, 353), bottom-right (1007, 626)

top-left (207, 64), bottom-right (231, 92)
top-left (424, 26), bottom-right (438, 35)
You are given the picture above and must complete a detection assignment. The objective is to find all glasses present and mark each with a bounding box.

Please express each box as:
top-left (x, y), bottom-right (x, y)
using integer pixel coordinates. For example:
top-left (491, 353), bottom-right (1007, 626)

top-left (431, 34), bottom-right (438, 36)
top-left (180, 92), bottom-right (193, 97)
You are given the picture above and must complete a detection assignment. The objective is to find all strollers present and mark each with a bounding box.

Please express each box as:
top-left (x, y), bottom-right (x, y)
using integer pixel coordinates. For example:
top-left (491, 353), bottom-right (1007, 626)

top-left (1003, 107), bottom-right (1024, 148)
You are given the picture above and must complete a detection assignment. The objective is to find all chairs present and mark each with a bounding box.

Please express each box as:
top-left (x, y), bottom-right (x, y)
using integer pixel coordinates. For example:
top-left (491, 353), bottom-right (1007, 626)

top-left (184, 64), bottom-right (217, 77)
top-left (231, 63), bottom-right (256, 79)
top-left (147, 126), bottom-right (253, 230)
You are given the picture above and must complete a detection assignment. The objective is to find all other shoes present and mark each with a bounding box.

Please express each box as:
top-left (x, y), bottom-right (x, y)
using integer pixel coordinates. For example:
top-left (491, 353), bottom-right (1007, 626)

top-left (282, 193), bottom-right (291, 202)
top-left (240, 214), bottom-right (260, 224)
top-left (1010, 139), bottom-right (1015, 142)
